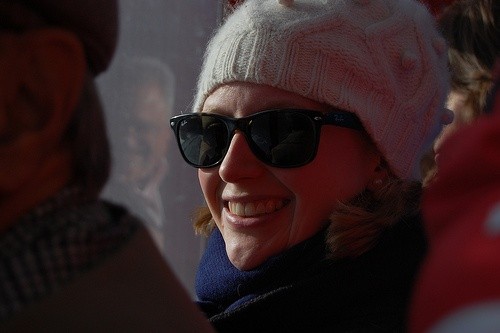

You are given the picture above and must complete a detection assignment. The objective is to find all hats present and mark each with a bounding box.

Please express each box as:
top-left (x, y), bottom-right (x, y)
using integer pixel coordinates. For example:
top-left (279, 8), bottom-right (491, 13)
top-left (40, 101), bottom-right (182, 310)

top-left (192, 0), bottom-right (454, 180)
top-left (0, 0), bottom-right (119, 76)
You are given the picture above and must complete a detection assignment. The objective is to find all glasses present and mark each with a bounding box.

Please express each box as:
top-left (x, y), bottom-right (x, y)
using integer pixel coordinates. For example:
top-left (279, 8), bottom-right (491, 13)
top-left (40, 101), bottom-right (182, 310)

top-left (169, 108), bottom-right (362, 169)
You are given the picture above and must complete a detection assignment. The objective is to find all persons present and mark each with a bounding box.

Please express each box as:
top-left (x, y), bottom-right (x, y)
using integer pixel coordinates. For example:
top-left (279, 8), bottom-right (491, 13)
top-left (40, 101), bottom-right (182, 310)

top-left (170, 0), bottom-right (500, 333)
top-left (0, 0), bottom-right (215, 333)
